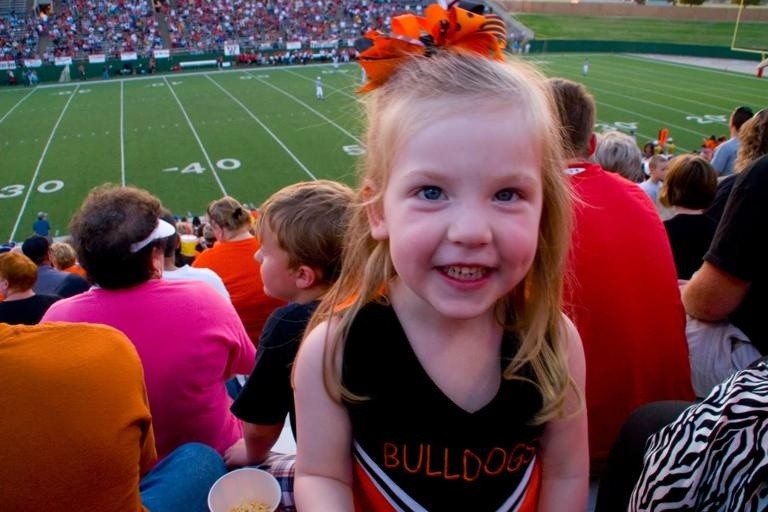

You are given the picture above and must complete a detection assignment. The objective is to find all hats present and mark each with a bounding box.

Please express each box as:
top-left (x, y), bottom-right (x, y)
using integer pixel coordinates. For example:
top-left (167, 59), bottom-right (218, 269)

top-left (130, 218), bottom-right (176, 256)
top-left (24, 237), bottom-right (54, 258)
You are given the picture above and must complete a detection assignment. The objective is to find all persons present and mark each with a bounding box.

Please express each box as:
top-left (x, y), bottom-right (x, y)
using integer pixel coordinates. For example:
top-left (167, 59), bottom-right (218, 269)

top-left (1, 1), bottom-right (434, 86)
top-left (613, 355), bottom-right (768, 512)
top-left (0, 323), bottom-right (225, 512)
top-left (544, 80), bottom-right (694, 484)
top-left (710, 106), bottom-right (754, 177)
top-left (22, 237), bottom-right (92, 299)
top-left (170, 216), bottom-right (215, 268)
top-left (38, 186), bottom-right (257, 471)
top-left (293, 51), bottom-right (589, 512)
top-left (33, 212), bottom-right (51, 238)
top-left (0, 252), bottom-right (64, 325)
top-left (223, 179), bottom-right (360, 469)
top-left (191, 198), bottom-right (288, 350)
top-left (158, 207), bottom-right (250, 401)
top-left (714, 108), bottom-right (768, 211)
top-left (52, 243), bottom-right (87, 277)
top-left (591, 131), bottom-right (727, 204)
top-left (660, 157), bottom-right (728, 283)
top-left (678, 154), bottom-right (768, 400)
